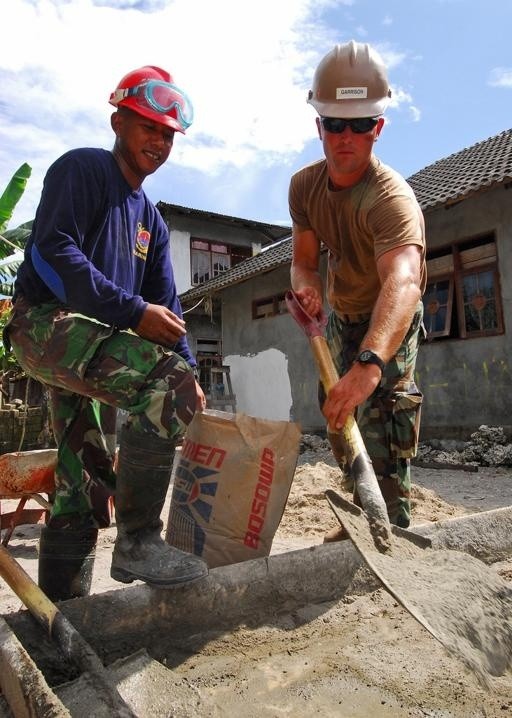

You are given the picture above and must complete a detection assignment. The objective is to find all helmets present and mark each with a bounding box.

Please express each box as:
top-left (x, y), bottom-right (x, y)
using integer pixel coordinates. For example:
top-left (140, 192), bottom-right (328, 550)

top-left (109, 66), bottom-right (193, 135)
top-left (306, 40), bottom-right (391, 120)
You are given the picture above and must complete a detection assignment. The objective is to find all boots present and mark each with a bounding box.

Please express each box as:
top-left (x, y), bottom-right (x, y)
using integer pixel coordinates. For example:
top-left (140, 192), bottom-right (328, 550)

top-left (324, 476), bottom-right (410, 541)
top-left (110, 424), bottom-right (208, 590)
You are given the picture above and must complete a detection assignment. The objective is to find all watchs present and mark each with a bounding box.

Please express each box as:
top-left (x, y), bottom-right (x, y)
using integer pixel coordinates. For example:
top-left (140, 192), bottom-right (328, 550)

top-left (349, 349), bottom-right (385, 374)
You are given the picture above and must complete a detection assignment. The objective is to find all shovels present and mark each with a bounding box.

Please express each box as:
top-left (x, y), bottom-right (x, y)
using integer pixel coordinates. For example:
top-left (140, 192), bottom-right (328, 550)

top-left (284, 288), bottom-right (507, 678)
top-left (0, 543), bottom-right (207, 718)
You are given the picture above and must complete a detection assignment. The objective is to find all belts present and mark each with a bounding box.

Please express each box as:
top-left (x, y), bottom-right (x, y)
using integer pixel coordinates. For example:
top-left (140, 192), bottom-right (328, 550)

top-left (334, 311), bottom-right (369, 324)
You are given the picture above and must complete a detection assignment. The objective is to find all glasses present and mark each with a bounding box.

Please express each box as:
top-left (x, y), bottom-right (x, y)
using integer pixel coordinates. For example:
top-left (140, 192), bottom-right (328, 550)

top-left (321, 118), bottom-right (378, 133)
top-left (144, 79), bottom-right (193, 129)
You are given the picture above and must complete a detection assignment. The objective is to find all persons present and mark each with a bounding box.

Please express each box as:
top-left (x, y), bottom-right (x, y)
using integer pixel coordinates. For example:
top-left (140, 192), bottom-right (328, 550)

top-left (288, 40), bottom-right (429, 545)
top-left (1, 62), bottom-right (212, 593)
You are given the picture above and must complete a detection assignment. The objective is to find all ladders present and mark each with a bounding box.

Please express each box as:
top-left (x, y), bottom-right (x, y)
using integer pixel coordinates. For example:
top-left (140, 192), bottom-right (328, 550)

top-left (206, 365), bottom-right (237, 414)
